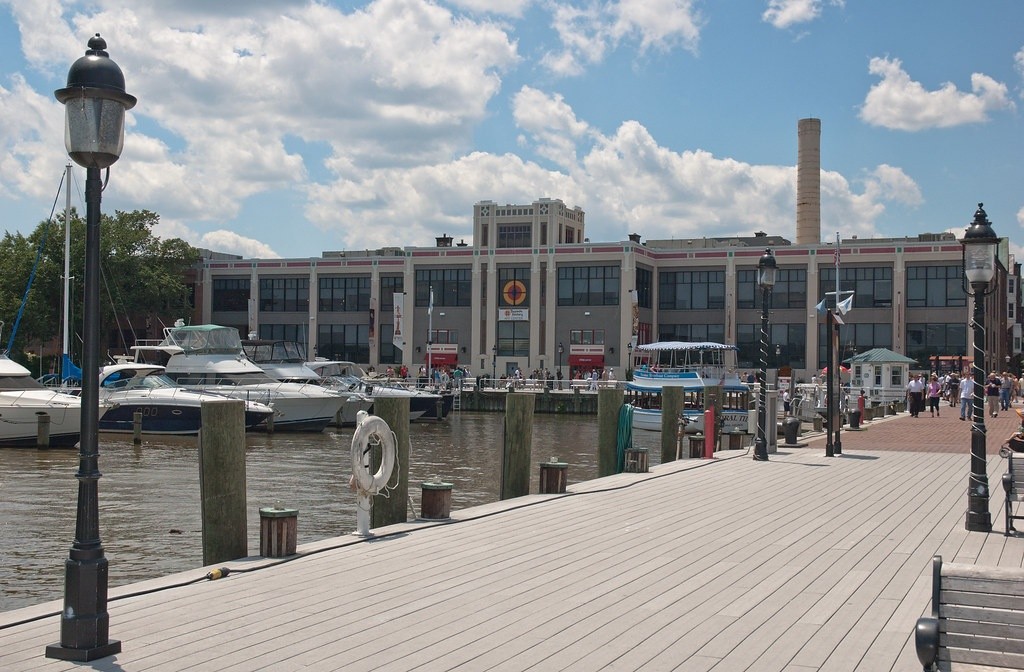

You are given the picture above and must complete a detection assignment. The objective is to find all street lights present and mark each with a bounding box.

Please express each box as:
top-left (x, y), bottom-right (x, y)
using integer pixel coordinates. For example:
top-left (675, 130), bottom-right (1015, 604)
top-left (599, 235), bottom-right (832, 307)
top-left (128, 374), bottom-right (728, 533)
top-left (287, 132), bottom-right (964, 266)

top-left (955, 201), bottom-right (1005, 533)
top-left (752, 247), bottom-right (780, 462)
top-left (558, 343), bottom-right (564, 389)
top-left (626, 342), bottom-right (632, 380)
top-left (43, 32), bottom-right (123, 662)
top-left (492, 345), bottom-right (498, 389)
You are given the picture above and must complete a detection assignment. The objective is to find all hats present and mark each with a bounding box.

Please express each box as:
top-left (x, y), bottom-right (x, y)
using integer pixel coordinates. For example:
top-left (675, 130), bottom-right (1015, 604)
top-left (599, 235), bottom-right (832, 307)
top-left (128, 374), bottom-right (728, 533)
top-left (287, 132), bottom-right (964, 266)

top-left (610, 368), bottom-right (613, 370)
top-left (950, 373), bottom-right (956, 376)
top-left (1016, 408), bottom-right (1024, 417)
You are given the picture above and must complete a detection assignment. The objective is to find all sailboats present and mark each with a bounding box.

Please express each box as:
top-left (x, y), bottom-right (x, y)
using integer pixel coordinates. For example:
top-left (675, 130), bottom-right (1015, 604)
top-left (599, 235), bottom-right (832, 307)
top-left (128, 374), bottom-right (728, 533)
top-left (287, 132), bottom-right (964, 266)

top-left (0, 157), bottom-right (275, 438)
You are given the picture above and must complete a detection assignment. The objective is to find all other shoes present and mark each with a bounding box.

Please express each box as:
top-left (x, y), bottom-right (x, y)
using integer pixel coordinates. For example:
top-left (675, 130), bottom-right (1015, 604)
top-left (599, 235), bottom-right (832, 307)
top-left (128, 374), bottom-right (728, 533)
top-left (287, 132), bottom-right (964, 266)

top-left (949, 404), bottom-right (953, 407)
top-left (915, 415), bottom-right (918, 418)
top-left (937, 411), bottom-right (939, 417)
top-left (967, 416), bottom-right (972, 421)
top-left (932, 413), bottom-right (934, 417)
top-left (1001, 407), bottom-right (1004, 411)
top-left (994, 413), bottom-right (997, 418)
top-left (1005, 408), bottom-right (1008, 411)
top-left (959, 417), bottom-right (965, 421)
top-left (910, 414), bottom-right (914, 416)
top-left (990, 415), bottom-right (993, 417)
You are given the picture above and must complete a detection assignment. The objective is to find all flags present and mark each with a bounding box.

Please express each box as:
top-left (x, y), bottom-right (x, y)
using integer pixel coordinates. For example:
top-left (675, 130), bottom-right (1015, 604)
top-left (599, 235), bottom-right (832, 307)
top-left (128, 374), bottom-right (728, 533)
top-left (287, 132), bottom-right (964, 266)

top-left (838, 294), bottom-right (853, 315)
top-left (815, 297), bottom-right (827, 312)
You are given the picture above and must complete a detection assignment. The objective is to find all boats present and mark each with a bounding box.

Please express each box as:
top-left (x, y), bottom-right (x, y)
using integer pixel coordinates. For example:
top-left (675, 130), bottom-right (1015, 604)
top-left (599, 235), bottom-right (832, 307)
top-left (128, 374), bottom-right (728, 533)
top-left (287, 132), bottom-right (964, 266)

top-left (0, 348), bottom-right (113, 451)
top-left (625, 339), bottom-right (757, 435)
top-left (113, 313), bottom-right (455, 434)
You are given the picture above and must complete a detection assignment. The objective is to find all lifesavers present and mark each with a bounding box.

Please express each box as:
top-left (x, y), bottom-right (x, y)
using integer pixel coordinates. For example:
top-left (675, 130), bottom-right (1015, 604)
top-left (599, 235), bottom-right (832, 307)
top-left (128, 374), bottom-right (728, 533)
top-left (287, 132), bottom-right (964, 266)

top-left (351, 415), bottom-right (396, 494)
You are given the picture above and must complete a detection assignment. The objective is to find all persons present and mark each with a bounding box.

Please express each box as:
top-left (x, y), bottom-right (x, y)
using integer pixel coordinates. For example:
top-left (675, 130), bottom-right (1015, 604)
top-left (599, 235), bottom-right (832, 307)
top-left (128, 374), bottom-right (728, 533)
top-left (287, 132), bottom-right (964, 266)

top-left (370, 365), bottom-right (375, 372)
top-left (906, 372), bottom-right (1024, 421)
top-left (386, 364), bottom-right (409, 386)
top-left (416, 365), bottom-right (473, 390)
top-left (782, 388), bottom-right (790, 414)
top-left (532, 368), bottom-right (563, 389)
top-left (498, 367), bottom-right (526, 389)
top-left (741, 370), bottom-right (758, 389)
top-left (811, 374), bottom-right (822, 384)
top-left (574, 368), bottom-right (615, 391)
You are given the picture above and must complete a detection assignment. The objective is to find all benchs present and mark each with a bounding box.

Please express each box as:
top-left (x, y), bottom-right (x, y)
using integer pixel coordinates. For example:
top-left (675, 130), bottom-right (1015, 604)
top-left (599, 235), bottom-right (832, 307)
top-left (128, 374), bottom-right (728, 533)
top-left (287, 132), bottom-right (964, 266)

top-left (1002, 451), bottom-right (1024, 535)
top-left (915, 555), bottom-right (1024, 672)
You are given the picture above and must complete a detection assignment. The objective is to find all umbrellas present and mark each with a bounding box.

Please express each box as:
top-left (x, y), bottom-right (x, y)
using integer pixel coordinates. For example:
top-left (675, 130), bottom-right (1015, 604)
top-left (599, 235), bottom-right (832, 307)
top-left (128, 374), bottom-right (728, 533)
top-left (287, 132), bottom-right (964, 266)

top-left (822, 365), bottom-right (847, 374)
